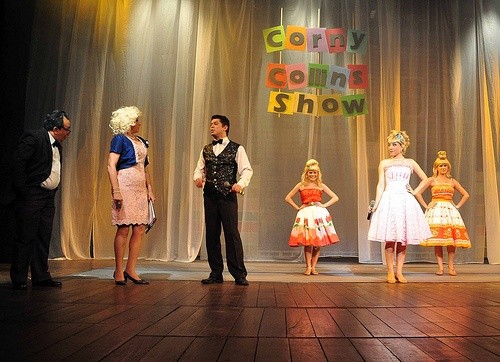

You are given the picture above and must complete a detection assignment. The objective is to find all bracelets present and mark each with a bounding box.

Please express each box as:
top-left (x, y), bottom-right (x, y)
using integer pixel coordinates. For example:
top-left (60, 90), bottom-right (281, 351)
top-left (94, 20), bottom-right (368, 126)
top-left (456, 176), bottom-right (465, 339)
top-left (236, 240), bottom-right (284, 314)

top-left (113, 191), bottom-right (120, 193)
top-left (113, 187), bottom-right (119, 189)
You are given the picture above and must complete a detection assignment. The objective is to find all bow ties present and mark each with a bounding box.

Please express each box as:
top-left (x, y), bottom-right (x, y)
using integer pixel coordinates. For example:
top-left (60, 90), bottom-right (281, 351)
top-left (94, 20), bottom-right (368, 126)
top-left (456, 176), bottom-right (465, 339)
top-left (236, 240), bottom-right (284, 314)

top-left (52, 141), bottom-right (60, 147)
top-left (212, 139), bottom-right (223, 145)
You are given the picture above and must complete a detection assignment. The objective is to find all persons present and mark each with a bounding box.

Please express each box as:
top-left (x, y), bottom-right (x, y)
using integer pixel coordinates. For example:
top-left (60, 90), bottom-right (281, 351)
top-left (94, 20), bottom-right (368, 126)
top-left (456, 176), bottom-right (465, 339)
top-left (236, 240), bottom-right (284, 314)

top-left (416, 150), bottom-right (471, 276)
top-left (107, 106), bottom-right (155, 285)
top-left (285, 159), bottom-right (339, 276)
top-left (193, 115), bottom-right (253, 286)
top-left (368, 130), bottom-right (433, 284)
top-left (5, 109), bottom-right (72, 289)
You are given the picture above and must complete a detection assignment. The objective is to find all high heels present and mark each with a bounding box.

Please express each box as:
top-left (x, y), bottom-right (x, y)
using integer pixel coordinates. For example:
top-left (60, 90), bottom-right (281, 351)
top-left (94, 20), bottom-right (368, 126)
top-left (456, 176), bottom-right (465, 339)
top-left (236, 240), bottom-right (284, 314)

top-left (113, 270), bottom-right (127, 285)
top-left (124, 271), bottom-right (149, 284)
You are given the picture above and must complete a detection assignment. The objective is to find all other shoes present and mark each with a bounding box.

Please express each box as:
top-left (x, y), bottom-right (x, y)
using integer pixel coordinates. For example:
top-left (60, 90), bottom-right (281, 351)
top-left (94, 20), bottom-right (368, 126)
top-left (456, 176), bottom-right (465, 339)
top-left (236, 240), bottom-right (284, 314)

top-left (436, 267), bottom-right (444, 275)
top-left (448, 265), bottom-right (456, 276)
top-left (31, 279), bottom-right (62, 288)
top-left (304, 267), bottom-right (318, 276)
top-left (387, 274), bottom-right (407, 284)
top-left (201, 276), bottom-right (223, 284)
top-left (12, 280), bottom-right (28, 289)
top-left (235, 278), bottom-right (248, 285)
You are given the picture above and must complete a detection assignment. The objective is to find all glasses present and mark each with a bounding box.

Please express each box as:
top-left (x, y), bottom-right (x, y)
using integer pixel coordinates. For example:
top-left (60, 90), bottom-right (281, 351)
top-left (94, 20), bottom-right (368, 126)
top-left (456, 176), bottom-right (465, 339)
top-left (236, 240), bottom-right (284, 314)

top-left (61, 125), bottom-right (71, 132)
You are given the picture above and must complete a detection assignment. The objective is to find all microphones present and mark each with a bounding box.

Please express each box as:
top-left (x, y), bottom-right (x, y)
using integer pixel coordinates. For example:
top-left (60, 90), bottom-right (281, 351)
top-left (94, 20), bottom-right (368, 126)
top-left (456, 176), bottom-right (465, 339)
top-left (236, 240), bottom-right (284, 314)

top-left (367, 200), bottom-right (375, 220)
top-left (223, 181), bottom-right (245, 196)
